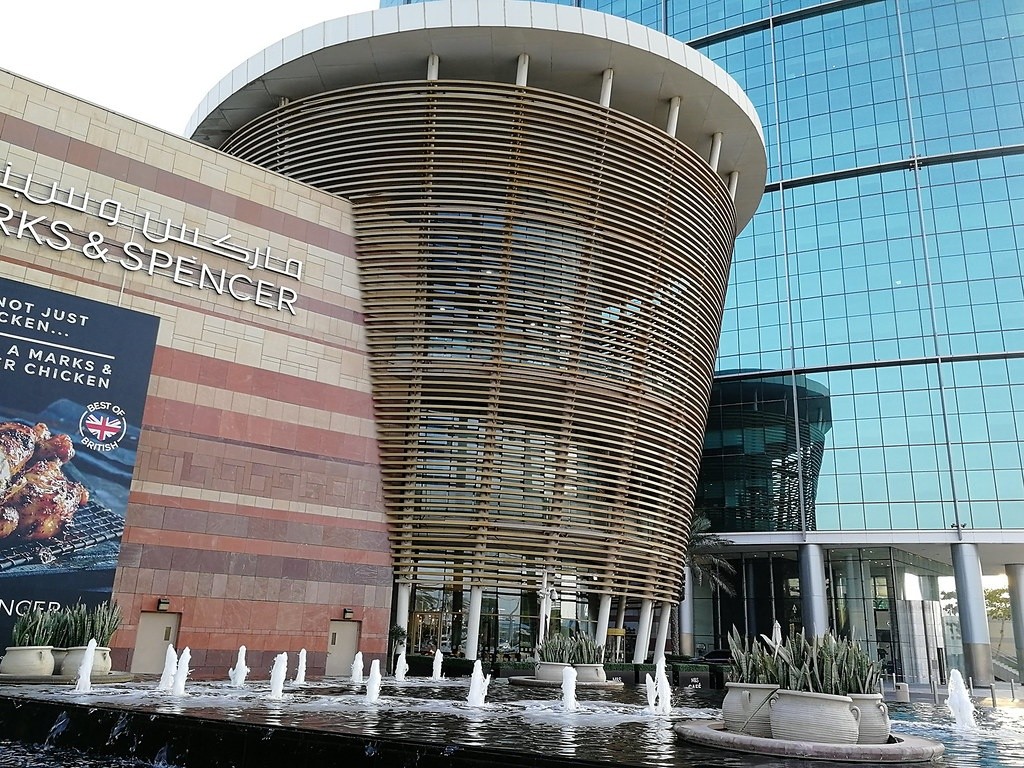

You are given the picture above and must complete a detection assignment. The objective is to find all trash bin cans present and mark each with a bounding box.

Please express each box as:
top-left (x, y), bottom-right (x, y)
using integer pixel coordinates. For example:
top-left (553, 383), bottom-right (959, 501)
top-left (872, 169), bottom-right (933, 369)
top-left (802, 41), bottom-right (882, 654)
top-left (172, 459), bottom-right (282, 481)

top-left (895, 683), bottom-right (909, 702)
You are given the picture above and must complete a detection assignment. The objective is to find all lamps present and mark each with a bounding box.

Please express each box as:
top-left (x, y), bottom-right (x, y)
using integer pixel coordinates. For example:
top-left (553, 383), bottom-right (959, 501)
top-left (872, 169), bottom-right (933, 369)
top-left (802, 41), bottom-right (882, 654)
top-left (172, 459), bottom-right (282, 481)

top-left (157, 599), bottom-right (170, 612)
top-left (344, 609), bottom-right (354, 619)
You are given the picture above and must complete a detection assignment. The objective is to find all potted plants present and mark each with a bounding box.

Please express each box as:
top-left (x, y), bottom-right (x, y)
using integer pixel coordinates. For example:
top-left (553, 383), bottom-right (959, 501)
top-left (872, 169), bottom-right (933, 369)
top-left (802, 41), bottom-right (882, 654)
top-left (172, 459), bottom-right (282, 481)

top-left (573, 630), bottom-right (606, 682)
top-left (534, 630), bottom-right (576, 680)
top-left (844, 634), bottom-right (892, 743)
top-left (718, 620), bottom-right (786, 737)
top-left (0, 601), bottom-right (126, 675)
top-left (769, 635), bottom-right (861, 748)
